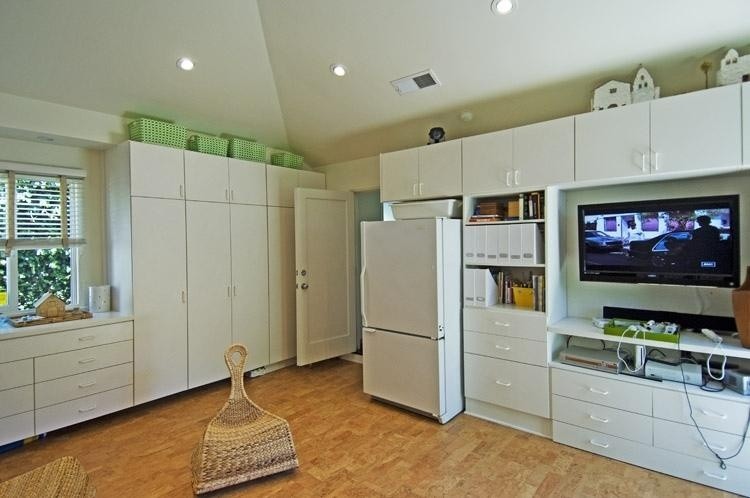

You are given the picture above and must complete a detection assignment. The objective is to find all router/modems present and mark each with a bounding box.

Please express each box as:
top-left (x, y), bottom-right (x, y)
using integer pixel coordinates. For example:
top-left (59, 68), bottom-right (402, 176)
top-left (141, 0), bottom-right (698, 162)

top-left (635, 344), bottom-right (646, 378)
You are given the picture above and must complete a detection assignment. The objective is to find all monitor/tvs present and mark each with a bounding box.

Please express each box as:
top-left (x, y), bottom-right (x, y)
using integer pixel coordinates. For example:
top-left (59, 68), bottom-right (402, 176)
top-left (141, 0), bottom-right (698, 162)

top-left (577, 194), bottom-right (740, 291)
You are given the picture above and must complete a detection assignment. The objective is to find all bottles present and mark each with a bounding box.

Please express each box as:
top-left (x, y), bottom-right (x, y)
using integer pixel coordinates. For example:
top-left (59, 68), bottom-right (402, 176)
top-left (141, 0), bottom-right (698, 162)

top-left (503, 273), bottom-right (514, 304)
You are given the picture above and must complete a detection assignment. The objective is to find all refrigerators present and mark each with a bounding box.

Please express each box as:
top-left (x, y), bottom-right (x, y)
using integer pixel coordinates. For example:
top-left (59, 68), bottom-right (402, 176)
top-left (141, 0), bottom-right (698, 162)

top-left (358, 216), bottom-right (464, 426)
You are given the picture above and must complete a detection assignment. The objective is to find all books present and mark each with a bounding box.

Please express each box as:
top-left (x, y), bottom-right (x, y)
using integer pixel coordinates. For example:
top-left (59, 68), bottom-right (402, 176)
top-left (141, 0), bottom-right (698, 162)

top-left (495, 271), bottom-right (545, 313)
top-left (468, 191), bottom-right (544, 222)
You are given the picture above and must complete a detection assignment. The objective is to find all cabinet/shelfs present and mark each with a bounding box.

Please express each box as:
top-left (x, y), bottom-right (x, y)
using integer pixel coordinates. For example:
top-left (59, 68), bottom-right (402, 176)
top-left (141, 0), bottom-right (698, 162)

top-left (460, 116), bottom-right (575, 196)
top-left (546, 365), bottom-right (750, 496)
top-left (456, 187), bottom-right (569, 441)
top-left (380, 136), bottom-right (461, 204)
top-left (103, 140), bottom-right (187, 406)
top-left (1, 322), bottom-right (135, 447)
top-left (267, 164), bottom-right (329, 360)
top-left (573, 83), bottom-right (744, 191)
top-left (184, 150), bottom-right (268, 394)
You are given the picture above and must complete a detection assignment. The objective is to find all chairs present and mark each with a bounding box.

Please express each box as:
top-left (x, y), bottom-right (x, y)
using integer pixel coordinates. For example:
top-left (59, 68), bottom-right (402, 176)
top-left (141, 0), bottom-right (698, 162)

top-left (188, 341), bottom-right (300, 498)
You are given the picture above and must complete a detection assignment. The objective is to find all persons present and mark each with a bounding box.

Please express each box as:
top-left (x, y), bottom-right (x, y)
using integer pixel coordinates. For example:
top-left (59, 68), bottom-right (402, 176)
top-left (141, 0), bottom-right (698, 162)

top-left (621, 222), bottom-right (644, 243)
top-left (692, 216), bottom-right (721, 242)
